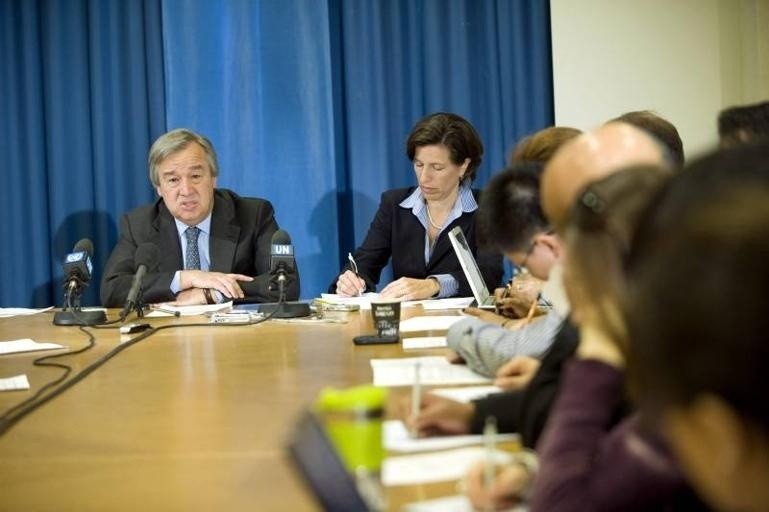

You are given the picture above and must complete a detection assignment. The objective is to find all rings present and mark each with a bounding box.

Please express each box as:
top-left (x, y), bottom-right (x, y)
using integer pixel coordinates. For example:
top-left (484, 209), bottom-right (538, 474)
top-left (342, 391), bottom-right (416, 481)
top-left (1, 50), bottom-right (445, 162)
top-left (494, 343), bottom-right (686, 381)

top-left (400, 288), bottom-right (404, 296)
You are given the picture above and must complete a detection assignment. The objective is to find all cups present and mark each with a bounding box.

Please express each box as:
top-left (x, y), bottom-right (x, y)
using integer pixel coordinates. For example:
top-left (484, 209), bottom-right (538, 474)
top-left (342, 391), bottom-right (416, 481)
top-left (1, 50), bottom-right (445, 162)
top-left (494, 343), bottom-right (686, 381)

top-left (315, 382), bottom-right (387, 485)
top-left (370, 300), bottom-right (400, 339)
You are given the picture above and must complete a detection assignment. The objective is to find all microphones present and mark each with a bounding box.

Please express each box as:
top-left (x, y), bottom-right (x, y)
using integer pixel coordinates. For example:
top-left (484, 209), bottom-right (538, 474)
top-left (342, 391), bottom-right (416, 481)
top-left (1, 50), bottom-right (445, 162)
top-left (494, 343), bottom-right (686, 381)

top-left (123, 242), bottom-right (161, 316)
top-left (269, 229), bottom-right (295, 289)
top-left (63, 239), bottom-right (94, 299)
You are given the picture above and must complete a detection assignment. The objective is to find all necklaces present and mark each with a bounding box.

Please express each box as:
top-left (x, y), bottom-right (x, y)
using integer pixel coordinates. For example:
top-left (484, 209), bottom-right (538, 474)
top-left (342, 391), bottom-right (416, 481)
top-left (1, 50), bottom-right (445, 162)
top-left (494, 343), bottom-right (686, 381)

top-left (425, 202), bottom-right (442, 231)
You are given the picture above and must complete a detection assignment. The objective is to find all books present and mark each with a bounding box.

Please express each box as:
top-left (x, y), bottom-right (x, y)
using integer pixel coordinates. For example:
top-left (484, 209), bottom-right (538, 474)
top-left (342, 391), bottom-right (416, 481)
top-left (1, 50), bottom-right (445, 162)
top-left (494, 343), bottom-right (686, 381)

top-left (311, 297), bottom-right (360, 312)
top-left (321, 291), bottom-right (378, 309)
top-left (211, 310), bottom-right (253, 324)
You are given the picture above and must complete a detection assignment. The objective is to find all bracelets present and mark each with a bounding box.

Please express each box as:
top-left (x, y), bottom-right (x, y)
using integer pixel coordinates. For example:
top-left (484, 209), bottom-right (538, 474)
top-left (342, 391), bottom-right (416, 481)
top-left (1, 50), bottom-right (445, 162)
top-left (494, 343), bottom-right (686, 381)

top-left (202, 287), bottom-right (217, 305)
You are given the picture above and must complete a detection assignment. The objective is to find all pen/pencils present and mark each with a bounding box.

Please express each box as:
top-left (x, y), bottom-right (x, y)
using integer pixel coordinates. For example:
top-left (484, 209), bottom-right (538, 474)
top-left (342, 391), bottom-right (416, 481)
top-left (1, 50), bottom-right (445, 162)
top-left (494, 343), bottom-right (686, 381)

top-left (484, 417), bottom-right (497, 487)
top-left (348, 252), bottom-right (363, 296)
top-left (410, 363), bottom-right (421, 439)
top-left (496, 279), bottom-right (512, 315)
top-left (146, 306), bottom-right (180, 316)
top-left (526, 293), bottom-right (541, 324)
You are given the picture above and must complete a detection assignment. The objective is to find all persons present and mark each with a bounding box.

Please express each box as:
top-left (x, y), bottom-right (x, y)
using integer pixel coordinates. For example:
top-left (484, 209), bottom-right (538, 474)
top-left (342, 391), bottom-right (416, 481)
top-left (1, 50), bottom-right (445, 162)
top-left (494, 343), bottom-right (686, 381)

top-left (385, 101), bottom-right (768, 512)
top-left (326, 110), bottom-right (506, 306)
top-left (99, 127), bottom-right (301, 307)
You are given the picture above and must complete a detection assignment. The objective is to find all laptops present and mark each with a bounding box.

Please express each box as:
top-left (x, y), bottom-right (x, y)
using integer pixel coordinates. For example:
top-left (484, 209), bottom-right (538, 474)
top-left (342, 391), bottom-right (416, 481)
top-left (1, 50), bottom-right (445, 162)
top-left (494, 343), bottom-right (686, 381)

top-left (448, 225), bottom-right (553, 313)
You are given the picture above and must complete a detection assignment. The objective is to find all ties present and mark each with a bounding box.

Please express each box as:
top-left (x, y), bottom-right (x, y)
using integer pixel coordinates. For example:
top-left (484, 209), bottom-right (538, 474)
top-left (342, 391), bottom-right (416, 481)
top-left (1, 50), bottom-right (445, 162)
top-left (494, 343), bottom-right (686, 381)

top-left (185, 226), bottom-right (201, 270)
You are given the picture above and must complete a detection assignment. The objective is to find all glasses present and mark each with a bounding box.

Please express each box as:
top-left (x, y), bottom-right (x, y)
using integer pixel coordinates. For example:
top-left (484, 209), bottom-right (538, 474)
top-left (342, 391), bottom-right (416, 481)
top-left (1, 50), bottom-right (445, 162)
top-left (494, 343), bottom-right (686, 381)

top-left (516, 228), bottom-right (554, 275)
